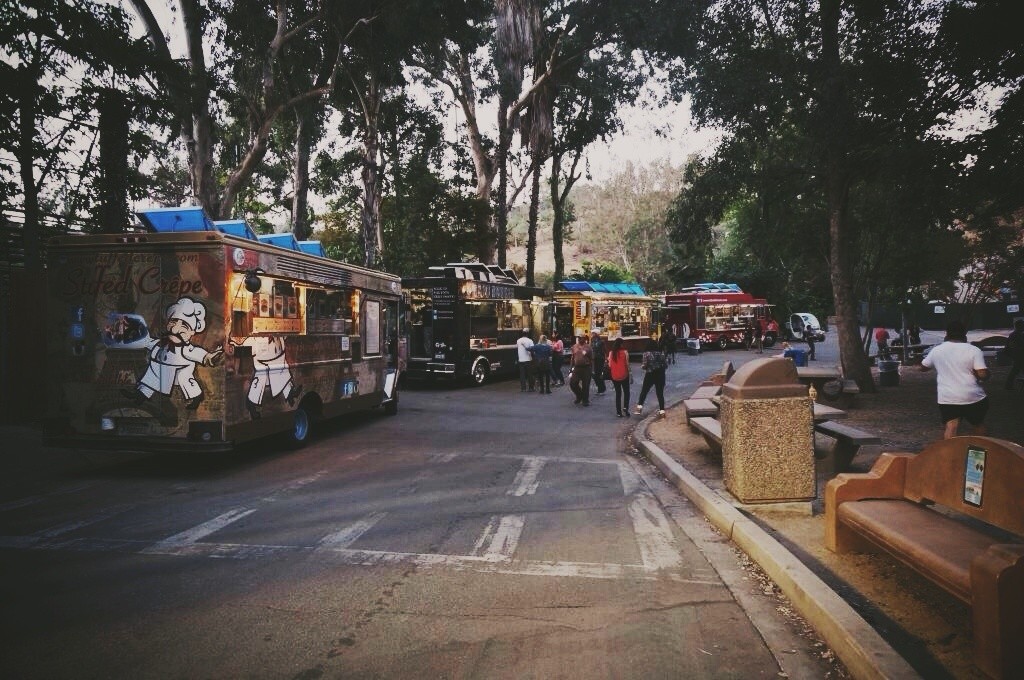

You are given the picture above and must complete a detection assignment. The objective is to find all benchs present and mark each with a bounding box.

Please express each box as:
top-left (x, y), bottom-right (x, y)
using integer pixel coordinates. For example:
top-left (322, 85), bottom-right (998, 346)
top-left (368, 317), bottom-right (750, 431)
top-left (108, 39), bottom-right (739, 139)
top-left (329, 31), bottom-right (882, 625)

top-left (870, 351), bottom-right (928, 365)
top-left (700, 360), bottom-right (736, 387)
top-left (841, 379), bottom-right (859, 401)
top-left (690, 385), bottom-right (722, 400)
top-left (971, 335), bottom-right (1009, 351)
top-left (690, 416), bottom-right (722, 455)
top-left (824, 435), bottom-right (1024, 680)
top-left (684, 398), bottom-right (719, 434)
top-left (815, 419), bottom-right (881, 473)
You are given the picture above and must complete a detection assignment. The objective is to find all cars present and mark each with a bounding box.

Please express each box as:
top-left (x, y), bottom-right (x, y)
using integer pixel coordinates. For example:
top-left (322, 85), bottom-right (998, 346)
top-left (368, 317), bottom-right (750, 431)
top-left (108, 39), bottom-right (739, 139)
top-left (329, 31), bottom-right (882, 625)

top-left (785, 313), bottom-right (826, 342)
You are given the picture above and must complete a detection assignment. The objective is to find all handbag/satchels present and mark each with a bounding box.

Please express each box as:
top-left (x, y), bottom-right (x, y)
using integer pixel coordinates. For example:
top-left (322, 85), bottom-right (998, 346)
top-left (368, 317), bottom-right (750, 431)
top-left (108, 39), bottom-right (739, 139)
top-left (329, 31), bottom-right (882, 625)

top-left (600, 364), bottom-right (612, 381)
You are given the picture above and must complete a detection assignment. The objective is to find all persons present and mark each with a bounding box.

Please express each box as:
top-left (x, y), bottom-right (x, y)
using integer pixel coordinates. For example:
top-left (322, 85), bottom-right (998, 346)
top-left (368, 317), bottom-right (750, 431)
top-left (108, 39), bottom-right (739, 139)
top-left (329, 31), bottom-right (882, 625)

top-left (891, 328), bottom-right (903, 346)
top-left (876, 327), bottom-right (892, 361)
top-left (516, 327), bottom-right (565, 395)
top-left (920, 318), bottom-right (992, 439)
top-left (745, 323), bottom-right (752, 351)
top-left (909, 324), bottom-right (923, 353)
top-left (805, 325), bottom-right (817, 361)
top-left (419, 297), bottom-right (433, 358)
top-left (1004, 320), bottom-right (1024, 392)
top-left (632, 340), bottom-right (668, 418)
top-left (754, 320), bottom-right (764, 354)
top-left (566, 332), bottom-right (634, 418)
top-left (660, 327), bottom-right (676, 364)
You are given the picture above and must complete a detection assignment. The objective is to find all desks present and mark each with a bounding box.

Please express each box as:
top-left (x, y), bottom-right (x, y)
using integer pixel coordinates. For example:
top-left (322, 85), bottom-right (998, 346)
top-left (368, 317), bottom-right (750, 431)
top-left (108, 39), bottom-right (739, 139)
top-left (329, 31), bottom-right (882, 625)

top-left (796, 365), bottom-right (842, 403)
top-left (812, 401), bottom-right (846, 429)
top-left (889, 343), bottom-right (934, 362)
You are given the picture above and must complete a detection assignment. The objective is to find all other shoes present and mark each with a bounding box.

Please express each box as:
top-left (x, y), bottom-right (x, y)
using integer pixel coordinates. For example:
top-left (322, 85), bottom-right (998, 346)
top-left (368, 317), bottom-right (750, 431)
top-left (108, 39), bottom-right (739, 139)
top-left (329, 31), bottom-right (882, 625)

top-left (599, 387), bottom-right (606, 393)
top-left (635, 408), bottom-right (641, 414)
top-left (584, 402), bottom-right (592, 407)
top-left (552, 381), bottom-right (557, 385)
top-left (623, 408), bottom-right (630, 416)
top-left (559, 380), bottom-right (565, 386)
top-left (659, 410), bottom-right (665, 418)
top-left (617, 412), bottom-right (622, 416)
top-left (575, 394), bottom-right (582, 403)
top-left (529, 388), bottom-right (536, 392)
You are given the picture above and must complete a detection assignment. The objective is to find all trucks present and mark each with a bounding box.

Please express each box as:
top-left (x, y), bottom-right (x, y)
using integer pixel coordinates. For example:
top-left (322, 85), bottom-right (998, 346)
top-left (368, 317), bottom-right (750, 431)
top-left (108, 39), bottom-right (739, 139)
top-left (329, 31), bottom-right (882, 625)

top-left (553, 279), bottom-right (667, 365)
top-left (662, 282), bottom-right (778, 350)
top-left (49, 206), bottom-right (411, 452)
top-left (398, 262), bottom-right (546, 389)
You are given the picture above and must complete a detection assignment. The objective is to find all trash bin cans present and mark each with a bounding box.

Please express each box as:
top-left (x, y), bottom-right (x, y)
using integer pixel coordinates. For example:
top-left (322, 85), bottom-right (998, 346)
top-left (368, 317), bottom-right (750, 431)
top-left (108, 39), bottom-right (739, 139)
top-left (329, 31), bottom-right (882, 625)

top-left (719, 357), bottom-right (817, 505)
top-left (784, 350), bottom-right (808, 367)
top-left (878, 361), bottom-right (901, 387)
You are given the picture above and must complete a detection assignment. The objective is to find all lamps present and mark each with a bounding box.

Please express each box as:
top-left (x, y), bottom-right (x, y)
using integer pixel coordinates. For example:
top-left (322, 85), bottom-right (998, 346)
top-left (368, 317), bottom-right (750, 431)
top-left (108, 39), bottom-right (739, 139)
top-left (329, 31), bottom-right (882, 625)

top-left (244, 267), bottom-right (263, 292)
